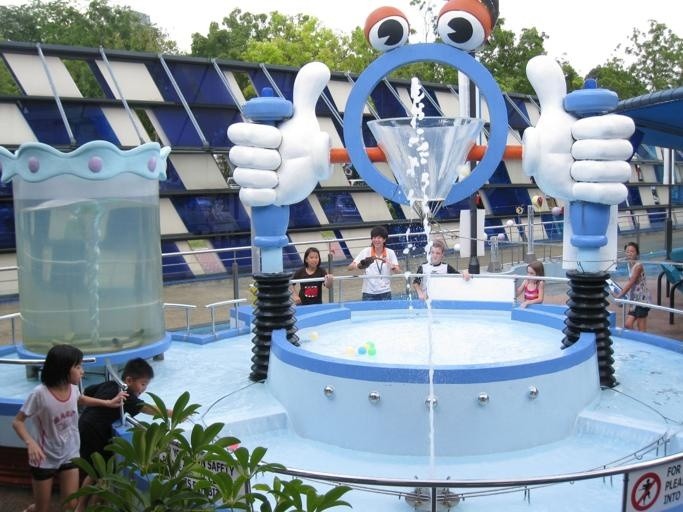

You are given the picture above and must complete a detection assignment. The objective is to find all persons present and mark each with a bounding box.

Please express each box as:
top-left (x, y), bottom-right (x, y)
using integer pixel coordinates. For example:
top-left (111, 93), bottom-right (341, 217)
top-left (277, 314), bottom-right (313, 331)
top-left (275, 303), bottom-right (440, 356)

top-left (73, 355), bottom-right (173, 511)
top-left (345, 226), bottom-right (400, 301)
top-left (516, 261), bottom-right (545, 310)
top-left (607, 242), bottom-right (651, 331)
top-left (11, 343), bottom-right (130, 511)
top-left (287, 247), bottom-right (334, 305)
top-left (412, 240), bottom-right (469, 300)
